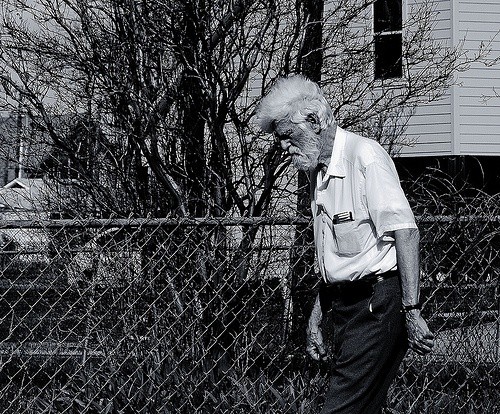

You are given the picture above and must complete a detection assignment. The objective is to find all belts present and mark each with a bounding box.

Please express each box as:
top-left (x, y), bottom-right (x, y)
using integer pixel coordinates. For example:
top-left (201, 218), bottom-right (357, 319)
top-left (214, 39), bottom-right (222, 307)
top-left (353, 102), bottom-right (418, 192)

top-left (319, 270), bottom-right (398, 307)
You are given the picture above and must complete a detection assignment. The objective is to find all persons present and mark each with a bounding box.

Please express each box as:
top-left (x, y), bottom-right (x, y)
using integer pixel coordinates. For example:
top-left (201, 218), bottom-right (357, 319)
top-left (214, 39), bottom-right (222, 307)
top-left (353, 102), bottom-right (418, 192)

top-left (254, 75), bottom-right (434, 414)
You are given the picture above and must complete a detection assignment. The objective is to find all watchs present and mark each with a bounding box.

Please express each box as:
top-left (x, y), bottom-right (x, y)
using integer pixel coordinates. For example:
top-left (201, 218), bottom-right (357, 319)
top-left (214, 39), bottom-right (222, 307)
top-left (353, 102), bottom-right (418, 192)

top-left (400, 303), bottom-right (421, 313)
